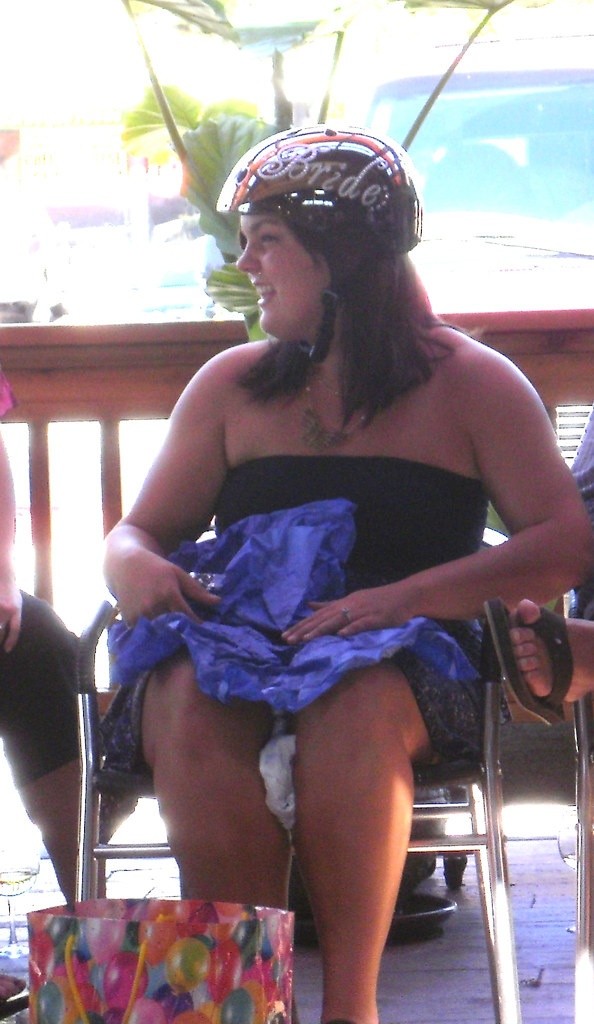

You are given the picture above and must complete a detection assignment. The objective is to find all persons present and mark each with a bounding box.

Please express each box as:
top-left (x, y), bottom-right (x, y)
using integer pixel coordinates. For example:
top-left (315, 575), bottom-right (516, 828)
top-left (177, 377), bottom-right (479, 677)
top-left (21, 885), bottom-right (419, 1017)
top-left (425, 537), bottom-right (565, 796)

top-left (481, 397), bottom-right (594, 735)
top-left (98, 127), bottom-right (593, 1024)
top-left (0, 384), bottom-right (108, 1006)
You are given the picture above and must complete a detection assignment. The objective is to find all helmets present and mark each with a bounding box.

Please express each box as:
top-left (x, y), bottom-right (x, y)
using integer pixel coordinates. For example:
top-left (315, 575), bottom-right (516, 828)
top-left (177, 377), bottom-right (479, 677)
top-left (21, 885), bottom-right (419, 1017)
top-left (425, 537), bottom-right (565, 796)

top-left (215, 123), bottom-right (424, 254)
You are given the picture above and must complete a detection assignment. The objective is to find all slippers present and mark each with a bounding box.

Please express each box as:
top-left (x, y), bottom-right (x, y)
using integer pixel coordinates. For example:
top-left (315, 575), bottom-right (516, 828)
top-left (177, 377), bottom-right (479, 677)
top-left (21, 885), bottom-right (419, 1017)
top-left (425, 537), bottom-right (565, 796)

top-left (0, 993), bottom-right (31, 1022)
top-left (482, 596), bottom-right (576, 728)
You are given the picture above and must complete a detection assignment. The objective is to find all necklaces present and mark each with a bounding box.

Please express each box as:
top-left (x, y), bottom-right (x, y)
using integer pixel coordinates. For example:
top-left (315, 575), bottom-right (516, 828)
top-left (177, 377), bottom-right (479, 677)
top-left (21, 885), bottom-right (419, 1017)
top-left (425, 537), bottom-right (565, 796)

top-left (301, 341), bottom-right (376, 450)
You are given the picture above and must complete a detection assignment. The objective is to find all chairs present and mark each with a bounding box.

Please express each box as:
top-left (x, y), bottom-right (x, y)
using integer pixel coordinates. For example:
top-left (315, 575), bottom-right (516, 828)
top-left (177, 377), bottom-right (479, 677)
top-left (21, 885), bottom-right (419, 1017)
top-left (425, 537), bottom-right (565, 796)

top-left (74, 518), bottom-right (524, 1024)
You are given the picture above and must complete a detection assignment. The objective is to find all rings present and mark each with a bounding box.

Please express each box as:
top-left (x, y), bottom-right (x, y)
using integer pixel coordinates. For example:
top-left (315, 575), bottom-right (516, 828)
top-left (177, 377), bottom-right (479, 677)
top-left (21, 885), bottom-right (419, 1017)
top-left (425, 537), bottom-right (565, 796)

top-left (340, 606), bottom-right (352, 622)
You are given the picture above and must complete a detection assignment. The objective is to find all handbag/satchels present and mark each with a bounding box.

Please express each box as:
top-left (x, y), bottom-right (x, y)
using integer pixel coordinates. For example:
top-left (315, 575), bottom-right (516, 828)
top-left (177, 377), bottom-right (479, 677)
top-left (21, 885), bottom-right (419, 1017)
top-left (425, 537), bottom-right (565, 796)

top-left (26, 897), bottom-right (296, 1024)
